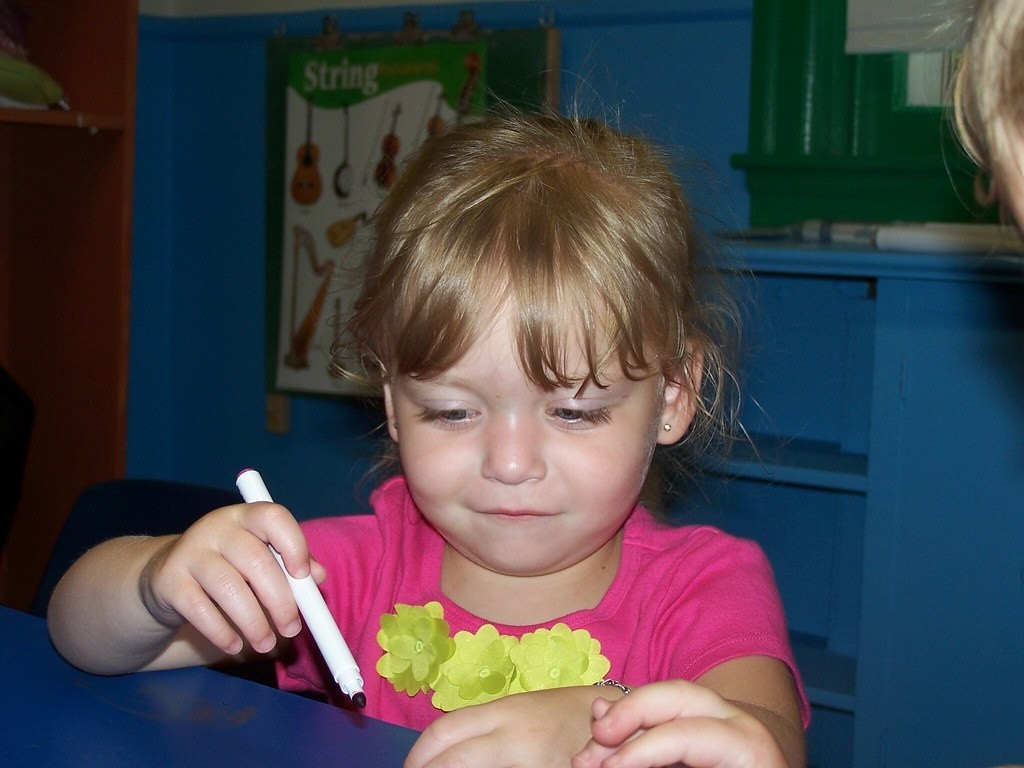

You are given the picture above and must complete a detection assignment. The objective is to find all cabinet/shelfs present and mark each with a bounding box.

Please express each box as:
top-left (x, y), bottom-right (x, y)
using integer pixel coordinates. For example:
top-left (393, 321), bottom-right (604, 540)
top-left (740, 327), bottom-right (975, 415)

top-left (0, 0), bottom-right (139, 614)
top-left (640, 235), bottom-right (1024, 768)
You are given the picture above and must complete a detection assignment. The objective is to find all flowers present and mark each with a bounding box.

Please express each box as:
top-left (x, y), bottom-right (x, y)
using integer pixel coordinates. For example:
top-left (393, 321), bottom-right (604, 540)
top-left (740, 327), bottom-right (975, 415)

top-left (377, 602), bottom-right (456, 696)
top-left (430, 624), bottom-right (520, 711)
top-left (510, 622), bottom-right (611, 692)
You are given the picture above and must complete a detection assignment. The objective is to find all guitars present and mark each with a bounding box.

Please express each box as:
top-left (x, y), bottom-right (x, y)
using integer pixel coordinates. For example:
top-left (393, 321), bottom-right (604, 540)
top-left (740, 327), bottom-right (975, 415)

top-left (288, 92), bottom-right (324, 208)
top-left (334, 103), bottom-right (356, 196)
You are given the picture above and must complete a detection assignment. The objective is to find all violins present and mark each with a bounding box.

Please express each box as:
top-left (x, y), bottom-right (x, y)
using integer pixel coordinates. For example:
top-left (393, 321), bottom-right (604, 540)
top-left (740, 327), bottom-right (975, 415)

top-left (376, 102), bottom-right (404, 187)
top-left (422, 89), bottom-right (449, 143)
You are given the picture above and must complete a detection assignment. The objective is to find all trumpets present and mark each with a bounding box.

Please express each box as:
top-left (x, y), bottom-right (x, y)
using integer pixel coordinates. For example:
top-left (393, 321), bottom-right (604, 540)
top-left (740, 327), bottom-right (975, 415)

top-left (327, 297), bottom-right (349, 377)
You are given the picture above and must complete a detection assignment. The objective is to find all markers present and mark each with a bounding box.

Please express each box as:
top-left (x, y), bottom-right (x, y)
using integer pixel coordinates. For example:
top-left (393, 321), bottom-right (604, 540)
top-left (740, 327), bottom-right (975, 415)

top-left (234, 468), bottom-right (367, 709)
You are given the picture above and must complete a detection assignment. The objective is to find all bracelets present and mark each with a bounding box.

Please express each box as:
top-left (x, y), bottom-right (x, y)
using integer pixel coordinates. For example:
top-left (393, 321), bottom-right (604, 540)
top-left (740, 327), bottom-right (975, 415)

top-left (593, 679), bottom-right (631, 696)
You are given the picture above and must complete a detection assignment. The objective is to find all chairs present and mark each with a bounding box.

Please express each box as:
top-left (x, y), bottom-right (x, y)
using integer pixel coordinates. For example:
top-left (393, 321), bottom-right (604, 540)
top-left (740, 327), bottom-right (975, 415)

top-left (30, 480), bottom-right (329, 705)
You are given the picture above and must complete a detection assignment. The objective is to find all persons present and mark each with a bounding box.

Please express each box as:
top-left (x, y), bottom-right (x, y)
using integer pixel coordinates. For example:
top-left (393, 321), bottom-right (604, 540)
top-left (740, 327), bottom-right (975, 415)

top-left (571, 0), bottom-right (1024, 767)
top-left (48, 102), bottom-right (813, 768)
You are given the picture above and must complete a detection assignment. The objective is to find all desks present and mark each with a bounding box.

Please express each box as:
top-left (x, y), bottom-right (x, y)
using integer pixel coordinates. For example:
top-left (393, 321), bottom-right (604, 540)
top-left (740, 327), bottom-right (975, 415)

top-left (0, 610), bottom-right (424, 768)
